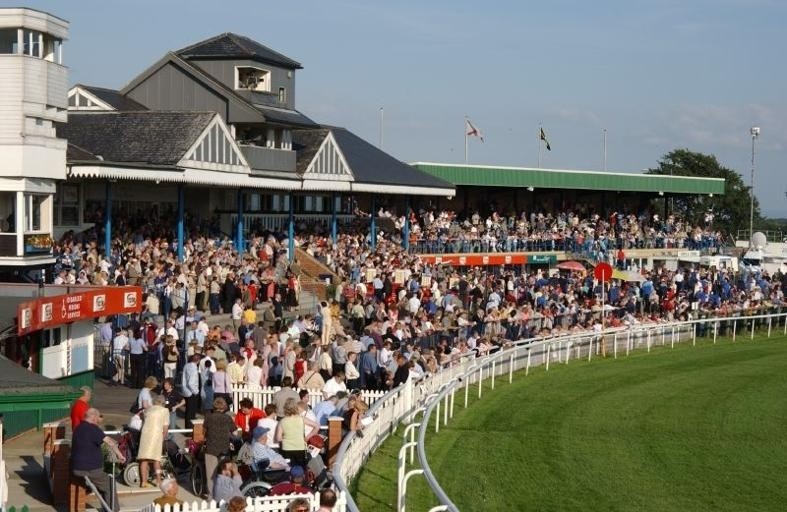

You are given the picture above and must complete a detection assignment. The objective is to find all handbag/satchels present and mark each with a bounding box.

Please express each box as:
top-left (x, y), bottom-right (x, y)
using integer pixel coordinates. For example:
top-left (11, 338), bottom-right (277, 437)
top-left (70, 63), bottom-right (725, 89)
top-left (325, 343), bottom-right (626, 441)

top-left (128, 399), bottom-right (143, 431)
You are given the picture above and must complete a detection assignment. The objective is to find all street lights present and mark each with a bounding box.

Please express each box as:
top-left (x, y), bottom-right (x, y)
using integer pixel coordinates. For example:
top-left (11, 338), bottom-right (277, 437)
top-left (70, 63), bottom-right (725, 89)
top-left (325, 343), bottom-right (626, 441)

top-left (600, 128), bottom-right (608, 172)
top-left (748, 126), bottom-right (761, 249)
top-left (377, 107), bottom-right (383, 148)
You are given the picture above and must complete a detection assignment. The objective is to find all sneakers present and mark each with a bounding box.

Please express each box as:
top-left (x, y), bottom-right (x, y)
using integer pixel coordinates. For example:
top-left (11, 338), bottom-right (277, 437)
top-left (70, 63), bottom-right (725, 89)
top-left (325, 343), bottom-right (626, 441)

top-left (141, 483), bottom-right (160, 488)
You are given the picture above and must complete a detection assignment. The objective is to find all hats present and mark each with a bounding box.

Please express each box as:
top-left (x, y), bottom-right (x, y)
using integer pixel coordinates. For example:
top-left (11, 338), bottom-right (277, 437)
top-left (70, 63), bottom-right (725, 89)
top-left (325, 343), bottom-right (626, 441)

top-left (253, 427), bottom-right (271, 438)
top-left (290, 466), bottom-right (303, 477)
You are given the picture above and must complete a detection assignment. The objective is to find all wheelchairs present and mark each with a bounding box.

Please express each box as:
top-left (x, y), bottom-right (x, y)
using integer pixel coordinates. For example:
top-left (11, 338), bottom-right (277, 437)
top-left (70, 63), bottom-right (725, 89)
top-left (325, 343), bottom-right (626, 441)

top-left (119, 424), bottom-right (177, 488)
top-left (184, 435), bottom-right (238, 498)
top-left (234, 455), bottom-right (275, 499)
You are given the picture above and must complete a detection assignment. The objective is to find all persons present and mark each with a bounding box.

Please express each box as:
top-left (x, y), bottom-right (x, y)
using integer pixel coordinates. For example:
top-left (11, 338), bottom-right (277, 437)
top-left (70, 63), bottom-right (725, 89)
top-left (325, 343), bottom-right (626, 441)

top-left (0, 196), bottom-right (786, 512)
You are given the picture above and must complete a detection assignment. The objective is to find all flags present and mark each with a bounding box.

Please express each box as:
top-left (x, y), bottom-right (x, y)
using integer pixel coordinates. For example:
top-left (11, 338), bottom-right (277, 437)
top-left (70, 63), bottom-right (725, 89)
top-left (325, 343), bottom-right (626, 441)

top-left (539, 128), bottom-right (551, 151)
top-left (463, 120), bottom-right (485, 142)
